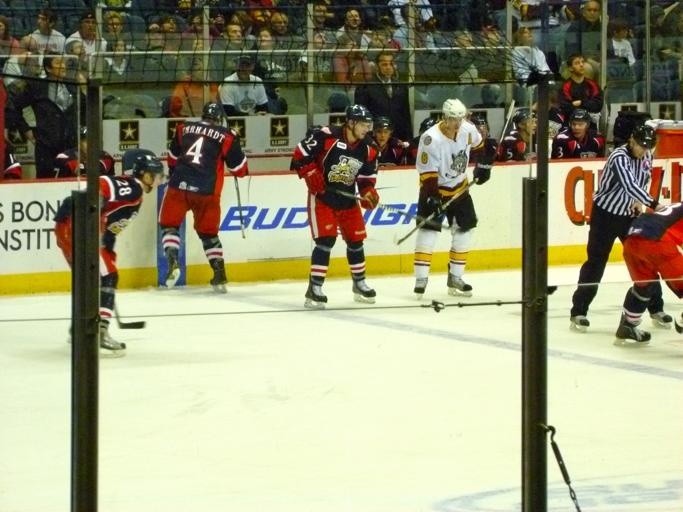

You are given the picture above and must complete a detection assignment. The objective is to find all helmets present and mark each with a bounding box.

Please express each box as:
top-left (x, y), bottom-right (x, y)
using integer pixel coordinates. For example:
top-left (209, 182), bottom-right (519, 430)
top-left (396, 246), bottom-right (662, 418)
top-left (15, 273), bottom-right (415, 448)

top-left (121, 150), bottom-right (163, 178)
top-left (80, 119), bottom-right (90, 143)
top-left (347, 99), bottom-right (592, 134)
top-left (633, 125), bottom-right (657, 150)
top-left (202, 102), bottom-right (228, 123)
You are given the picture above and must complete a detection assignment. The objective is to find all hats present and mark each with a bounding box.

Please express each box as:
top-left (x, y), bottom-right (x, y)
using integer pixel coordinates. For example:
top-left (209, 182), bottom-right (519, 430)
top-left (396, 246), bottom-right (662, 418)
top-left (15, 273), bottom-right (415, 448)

top-left (233, 55), bottom-right (255, 64)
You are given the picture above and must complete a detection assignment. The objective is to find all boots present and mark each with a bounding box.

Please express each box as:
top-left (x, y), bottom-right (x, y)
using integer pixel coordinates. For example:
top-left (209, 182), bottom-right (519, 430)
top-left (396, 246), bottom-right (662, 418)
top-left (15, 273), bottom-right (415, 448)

top-left (209, 258), bottom-right (228, 285)
top-left (166, 247), bottom-right (180, 287)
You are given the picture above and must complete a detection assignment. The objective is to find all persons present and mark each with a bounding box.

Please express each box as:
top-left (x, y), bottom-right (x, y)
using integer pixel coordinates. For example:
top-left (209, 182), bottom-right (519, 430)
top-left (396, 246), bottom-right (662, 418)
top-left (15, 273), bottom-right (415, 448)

top-left (51, 154), bottom-right (164, 359)
top-left (290, 103), bottom-right (379, 309)
top-left (1, 1), bottom-right (683, 178)
top-left (411, 98), bottom-right (490, 300)
top-left (569, 125), bottom-right (673, 334)
top-left (157, 100), bottom-right (249, 294)
top-left (613, 200), bottom-right (683, 345)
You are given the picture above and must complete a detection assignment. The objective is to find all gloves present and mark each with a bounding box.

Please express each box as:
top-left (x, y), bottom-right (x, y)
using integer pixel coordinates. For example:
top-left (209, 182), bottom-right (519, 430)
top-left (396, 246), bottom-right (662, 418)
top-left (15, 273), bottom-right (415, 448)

top-left (359, 187), bottom-right (379, 209)
top-left (304, 169), bottom-right (326, 195)
top-left (426, 197), bottom-right (442, 218)
top-left (473, 161), bottom-right (491, 184)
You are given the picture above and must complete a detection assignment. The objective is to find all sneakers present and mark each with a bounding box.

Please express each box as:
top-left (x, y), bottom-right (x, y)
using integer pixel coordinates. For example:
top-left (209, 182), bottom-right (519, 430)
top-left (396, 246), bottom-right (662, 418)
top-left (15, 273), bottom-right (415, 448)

top-left (303, 272), bottom-right (472, 302)
top-left (99, 330), bottom-right (126, 353)
top-left (570, 312), bottom-right (682, 342)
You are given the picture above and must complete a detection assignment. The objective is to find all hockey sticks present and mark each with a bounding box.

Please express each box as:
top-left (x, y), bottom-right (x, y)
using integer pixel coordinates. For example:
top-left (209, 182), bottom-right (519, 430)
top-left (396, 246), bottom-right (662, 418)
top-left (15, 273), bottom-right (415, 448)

top-left (233, 175), bottom-right (247, 239)
top-left (322, 185), bottom-right (460, 234)
top-left (391, 180), bottom-right (477, 246)
top-left (113, 302), bottom-right (144, 329)
